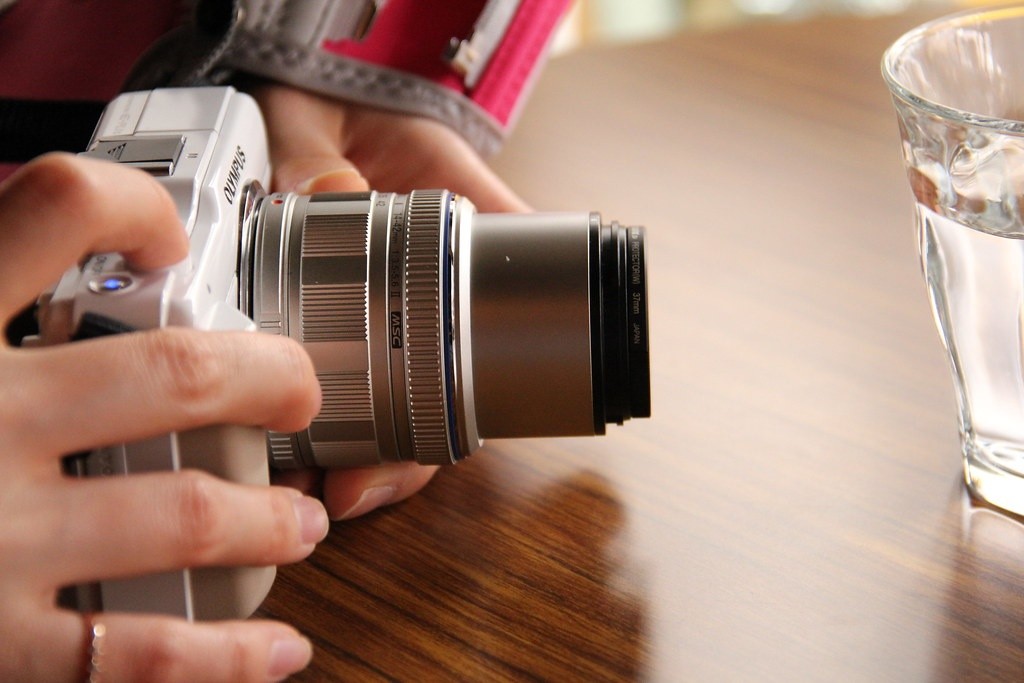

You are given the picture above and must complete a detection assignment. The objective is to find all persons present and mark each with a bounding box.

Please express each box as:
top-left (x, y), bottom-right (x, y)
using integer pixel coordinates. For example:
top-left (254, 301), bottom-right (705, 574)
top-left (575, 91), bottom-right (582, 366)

top-left (0, 0), bottom-right (577, 683)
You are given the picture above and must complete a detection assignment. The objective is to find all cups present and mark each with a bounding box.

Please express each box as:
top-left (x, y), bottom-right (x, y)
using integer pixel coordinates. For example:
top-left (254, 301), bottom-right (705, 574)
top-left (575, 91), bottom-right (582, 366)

top-left (880, 1), bottom-right (1024, 515)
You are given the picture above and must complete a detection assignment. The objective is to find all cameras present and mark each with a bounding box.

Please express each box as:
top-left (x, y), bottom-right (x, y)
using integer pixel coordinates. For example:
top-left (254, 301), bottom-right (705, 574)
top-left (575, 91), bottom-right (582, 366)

top-left (22, 83), bottom-right (651, 631)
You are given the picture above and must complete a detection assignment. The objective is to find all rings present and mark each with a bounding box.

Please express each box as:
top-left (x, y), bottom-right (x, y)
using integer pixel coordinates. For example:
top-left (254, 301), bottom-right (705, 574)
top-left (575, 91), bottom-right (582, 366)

top-left (82, 612), bottom-right (105, 683)
